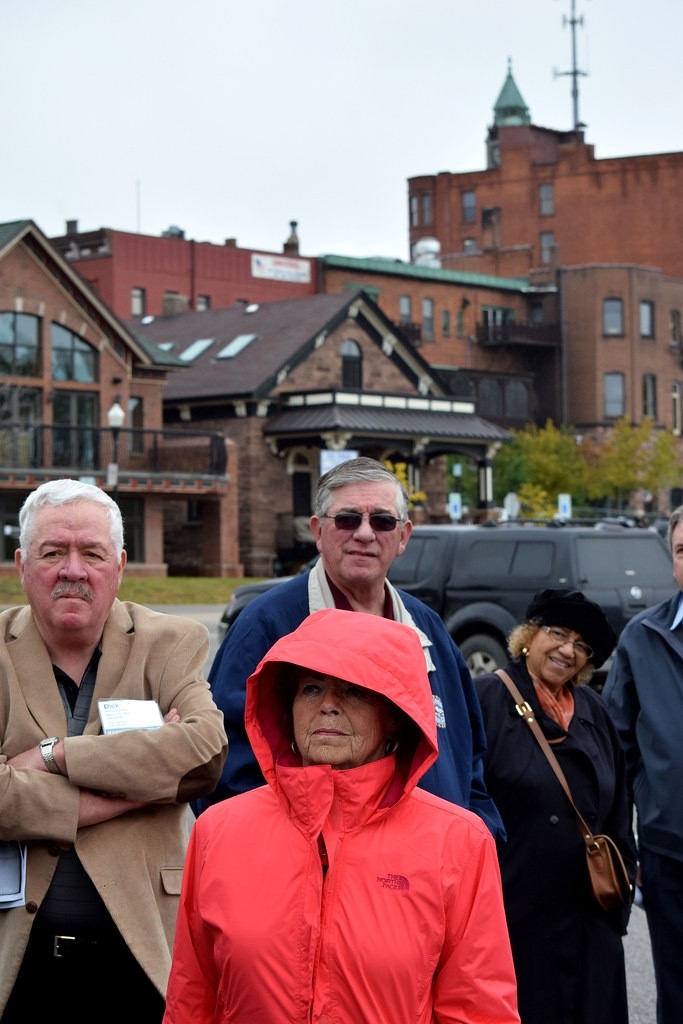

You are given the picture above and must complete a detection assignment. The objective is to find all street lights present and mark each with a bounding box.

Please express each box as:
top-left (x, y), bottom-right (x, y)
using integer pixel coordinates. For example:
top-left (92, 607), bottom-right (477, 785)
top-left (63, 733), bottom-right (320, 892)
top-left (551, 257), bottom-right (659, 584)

top-left (107, 404), bottom-right (127, 502)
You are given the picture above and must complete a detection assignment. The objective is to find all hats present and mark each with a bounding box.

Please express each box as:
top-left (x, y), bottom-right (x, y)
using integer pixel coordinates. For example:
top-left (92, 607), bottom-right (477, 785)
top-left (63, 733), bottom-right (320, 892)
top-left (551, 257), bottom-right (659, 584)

top-left (526, 588), bottom-right (619, 669)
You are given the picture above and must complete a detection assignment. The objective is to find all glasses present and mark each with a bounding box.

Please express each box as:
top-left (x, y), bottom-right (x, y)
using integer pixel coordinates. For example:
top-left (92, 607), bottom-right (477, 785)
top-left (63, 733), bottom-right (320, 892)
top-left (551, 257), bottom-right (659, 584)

top-left (321, 512), bottom-right (402, 531)
top-left (542, 626), bottom-right (594, 659)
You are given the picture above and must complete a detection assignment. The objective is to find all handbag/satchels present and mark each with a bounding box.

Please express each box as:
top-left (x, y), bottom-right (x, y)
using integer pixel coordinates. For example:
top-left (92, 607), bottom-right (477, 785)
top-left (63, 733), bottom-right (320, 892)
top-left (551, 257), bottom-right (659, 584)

top-left (587, 838), bottom-right (631, 927)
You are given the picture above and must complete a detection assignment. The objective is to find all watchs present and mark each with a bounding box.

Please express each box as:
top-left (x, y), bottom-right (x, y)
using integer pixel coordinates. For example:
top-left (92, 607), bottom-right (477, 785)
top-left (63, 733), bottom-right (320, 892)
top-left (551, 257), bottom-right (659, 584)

top-left (39, 736), bottom-right (63, 776)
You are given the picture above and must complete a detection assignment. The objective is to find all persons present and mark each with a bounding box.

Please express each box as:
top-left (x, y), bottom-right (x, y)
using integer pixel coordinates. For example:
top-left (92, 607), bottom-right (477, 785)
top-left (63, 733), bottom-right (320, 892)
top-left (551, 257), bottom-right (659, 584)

top-left (198, 457), bottom-right (513, 875)
top-left (1, 479), bottom-right (232, 1024)
top-left (155, 602), bottom-right (522, 1024)
top-left (599, 504), bottom-right (682, 1024)
top-left (478, 586), bottom-right (639, 1024)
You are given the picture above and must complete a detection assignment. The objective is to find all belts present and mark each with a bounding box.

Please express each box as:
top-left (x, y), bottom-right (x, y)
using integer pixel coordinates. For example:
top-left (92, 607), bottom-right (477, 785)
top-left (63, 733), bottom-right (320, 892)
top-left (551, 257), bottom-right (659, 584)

top-left (32, 928), bottom-right (119, 960)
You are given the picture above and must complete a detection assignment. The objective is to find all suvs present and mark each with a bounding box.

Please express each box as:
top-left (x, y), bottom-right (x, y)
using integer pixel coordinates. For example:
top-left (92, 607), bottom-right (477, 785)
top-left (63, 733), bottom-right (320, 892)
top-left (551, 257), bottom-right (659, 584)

top-left (216, 513), bottom-right (681, 706)
